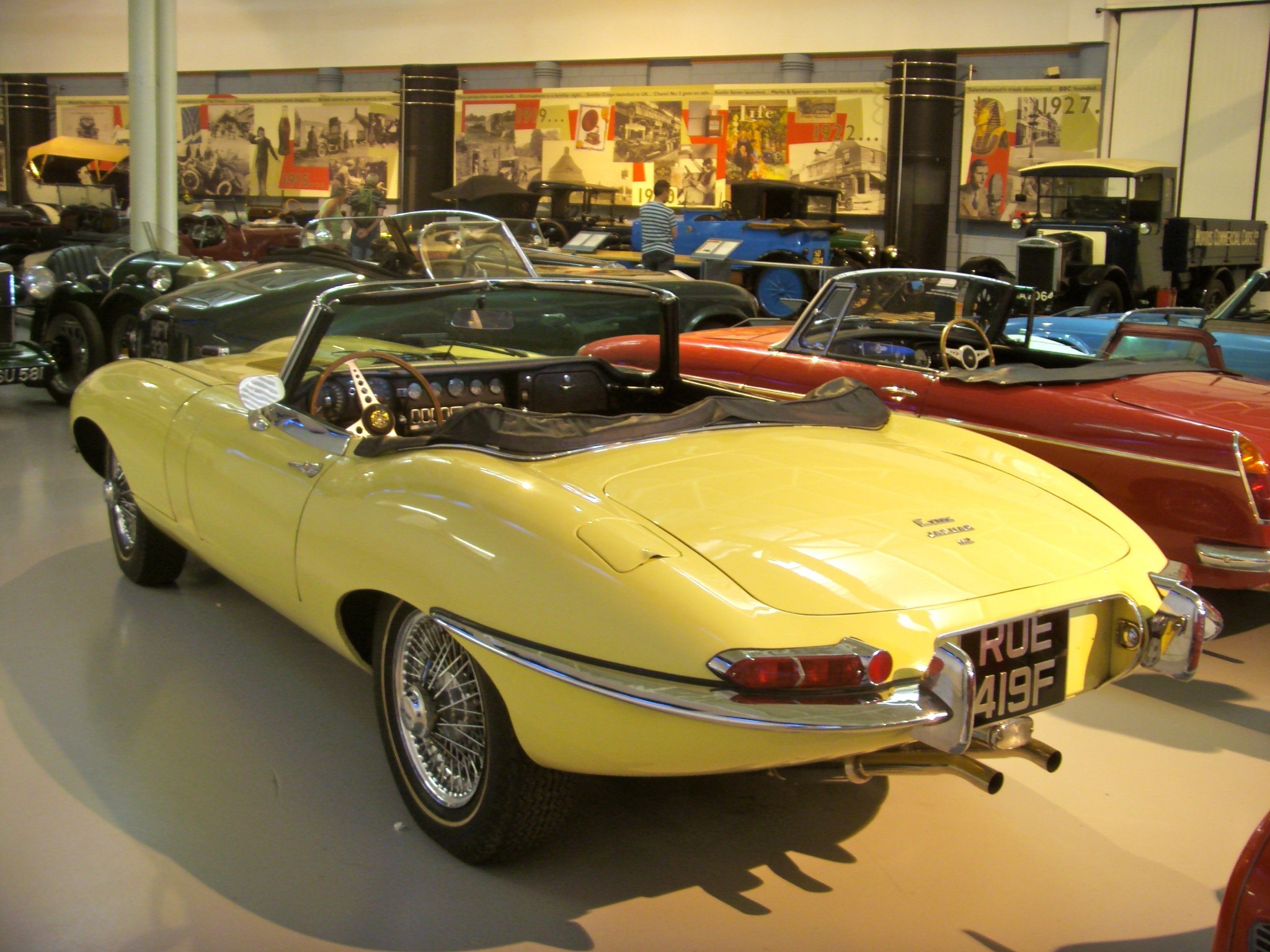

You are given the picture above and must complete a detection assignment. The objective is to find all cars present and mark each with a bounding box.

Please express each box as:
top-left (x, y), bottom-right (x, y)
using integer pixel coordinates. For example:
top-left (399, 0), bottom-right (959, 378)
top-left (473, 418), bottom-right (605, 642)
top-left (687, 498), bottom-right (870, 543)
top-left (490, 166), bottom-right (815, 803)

top-left (1, 179), bottom-right (1270, 867)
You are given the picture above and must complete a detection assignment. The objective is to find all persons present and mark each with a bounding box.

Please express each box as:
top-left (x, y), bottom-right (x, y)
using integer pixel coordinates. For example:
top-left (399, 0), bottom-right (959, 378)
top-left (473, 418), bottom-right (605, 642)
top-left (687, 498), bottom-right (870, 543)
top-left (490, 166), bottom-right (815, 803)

top-left (316, 187), bottom-right (346, 245)
top-left (347, 173), bottom-right (387, 261)
top-left (640, 179), bottom-right (678, 274)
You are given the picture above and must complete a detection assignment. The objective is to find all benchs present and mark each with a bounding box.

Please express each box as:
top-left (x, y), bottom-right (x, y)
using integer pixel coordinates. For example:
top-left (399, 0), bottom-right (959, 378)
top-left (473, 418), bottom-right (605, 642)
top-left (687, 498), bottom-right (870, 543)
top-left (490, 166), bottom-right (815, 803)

top-left (49, 245), bottom-right (133, 283)
top-left (1067, 196), bottom-right (1130, 220)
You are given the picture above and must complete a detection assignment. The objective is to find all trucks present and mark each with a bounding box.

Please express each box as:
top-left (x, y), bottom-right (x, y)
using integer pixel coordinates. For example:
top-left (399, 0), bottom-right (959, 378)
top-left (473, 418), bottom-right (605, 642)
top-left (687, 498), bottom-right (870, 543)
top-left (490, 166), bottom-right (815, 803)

top-left (957, 157), bottom-right (1268, 318)
